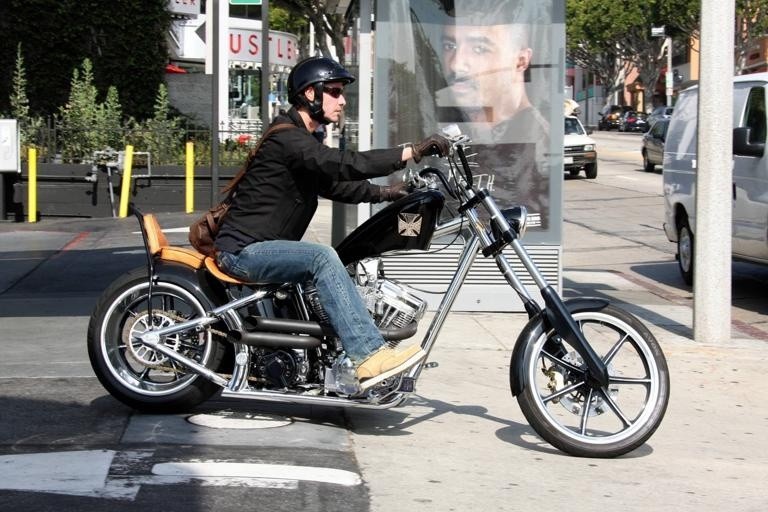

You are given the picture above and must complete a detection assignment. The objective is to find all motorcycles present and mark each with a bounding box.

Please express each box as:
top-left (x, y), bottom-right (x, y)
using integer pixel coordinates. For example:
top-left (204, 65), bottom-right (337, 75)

top-left (86, 121), bottom-right (672, 459)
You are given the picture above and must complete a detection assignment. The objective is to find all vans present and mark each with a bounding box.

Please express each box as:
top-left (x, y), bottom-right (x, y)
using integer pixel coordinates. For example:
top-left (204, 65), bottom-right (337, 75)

top-left (663, 70), bottom-right (768, 284)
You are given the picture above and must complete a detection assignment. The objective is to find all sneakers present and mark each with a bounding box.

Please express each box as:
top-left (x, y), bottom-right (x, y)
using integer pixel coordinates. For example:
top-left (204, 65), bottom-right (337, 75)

top-left (355, 344), bottom-right (427, 392)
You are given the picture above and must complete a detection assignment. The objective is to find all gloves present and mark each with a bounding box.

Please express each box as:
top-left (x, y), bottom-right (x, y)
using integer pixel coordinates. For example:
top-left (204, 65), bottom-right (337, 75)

top-left (412, 133), bottom-right (451, 163)
top-left (378, 182), bottom-right (411, 202)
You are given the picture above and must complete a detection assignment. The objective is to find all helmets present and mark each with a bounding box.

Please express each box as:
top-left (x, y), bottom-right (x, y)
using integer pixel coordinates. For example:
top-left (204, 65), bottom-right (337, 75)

top-left (287, 55), bottom-right (354, 101)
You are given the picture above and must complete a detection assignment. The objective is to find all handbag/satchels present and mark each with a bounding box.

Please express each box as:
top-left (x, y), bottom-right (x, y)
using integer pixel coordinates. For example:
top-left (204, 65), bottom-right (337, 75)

top-left (188, 124), bottom-right (298, 256)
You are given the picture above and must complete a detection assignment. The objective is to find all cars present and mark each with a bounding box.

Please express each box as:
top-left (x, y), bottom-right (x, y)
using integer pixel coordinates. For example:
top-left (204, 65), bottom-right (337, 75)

top-left (640, 118), bottom-right (672, 171)
top-left (617, 110), bottom-right (648, 133)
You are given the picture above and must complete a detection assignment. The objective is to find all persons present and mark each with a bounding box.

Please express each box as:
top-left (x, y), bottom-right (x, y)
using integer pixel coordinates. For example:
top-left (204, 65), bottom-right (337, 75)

top-left (209, 55), bottom-right (451, 393)
top-left (388, 0), bottom-right (550, 232)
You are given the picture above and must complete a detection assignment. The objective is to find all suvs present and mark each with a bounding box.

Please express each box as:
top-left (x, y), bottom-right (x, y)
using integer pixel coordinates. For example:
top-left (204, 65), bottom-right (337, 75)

top-left (597, 104), bottom-right (635, 130)
top-left (337, 123), bottom-right (358, 150)
top-left (645, 106), bottom-right (674, 127)
top-left (563, 110), bottom-right (598, 180)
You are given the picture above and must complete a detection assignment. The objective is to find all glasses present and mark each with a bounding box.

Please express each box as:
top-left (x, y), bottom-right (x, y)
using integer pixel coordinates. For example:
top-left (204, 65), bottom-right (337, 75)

top-left (322, 85), bottom-right (345, 98)
